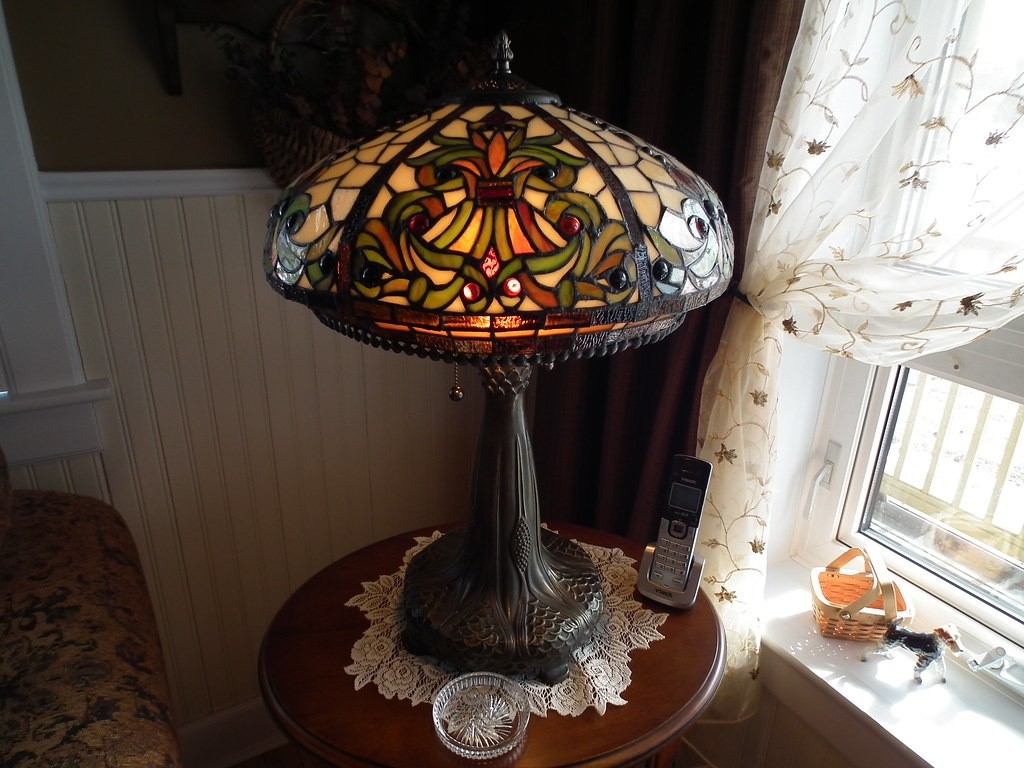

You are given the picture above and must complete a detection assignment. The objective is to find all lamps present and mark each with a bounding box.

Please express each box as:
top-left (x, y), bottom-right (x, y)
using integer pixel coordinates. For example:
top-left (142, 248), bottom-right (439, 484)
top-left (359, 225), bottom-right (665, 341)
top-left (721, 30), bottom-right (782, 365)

top-left (263, 30), bottom-right (735, 674)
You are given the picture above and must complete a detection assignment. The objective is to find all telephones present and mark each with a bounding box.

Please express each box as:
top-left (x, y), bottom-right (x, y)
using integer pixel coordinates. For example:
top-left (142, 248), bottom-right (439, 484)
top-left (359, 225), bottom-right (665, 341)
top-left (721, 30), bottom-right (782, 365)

top-left (633, 452), bottom-right (716, 608)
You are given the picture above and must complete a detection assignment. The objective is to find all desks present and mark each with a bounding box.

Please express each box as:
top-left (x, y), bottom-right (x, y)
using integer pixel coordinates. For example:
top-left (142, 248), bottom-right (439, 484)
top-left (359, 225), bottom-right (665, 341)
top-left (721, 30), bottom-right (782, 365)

top-left (255, 506), bottom-right (734, 766)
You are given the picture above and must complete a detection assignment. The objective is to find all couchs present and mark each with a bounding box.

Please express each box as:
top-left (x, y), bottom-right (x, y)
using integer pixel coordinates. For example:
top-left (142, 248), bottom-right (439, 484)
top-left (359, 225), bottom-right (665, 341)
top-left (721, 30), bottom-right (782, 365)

top-left (0, 444), bottom-right (188, 768)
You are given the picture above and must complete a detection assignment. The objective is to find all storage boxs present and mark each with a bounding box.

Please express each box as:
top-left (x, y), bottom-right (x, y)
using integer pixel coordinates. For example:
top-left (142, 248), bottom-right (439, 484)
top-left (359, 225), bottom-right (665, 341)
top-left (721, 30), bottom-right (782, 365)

top-left (809, 543), bottom-right (912, 643)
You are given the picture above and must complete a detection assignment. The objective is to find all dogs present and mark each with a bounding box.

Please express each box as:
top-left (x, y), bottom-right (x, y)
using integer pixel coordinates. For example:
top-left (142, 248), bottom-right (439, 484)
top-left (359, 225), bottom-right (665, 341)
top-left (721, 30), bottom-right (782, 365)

top-left (857, 615), bottom-right (966, 685)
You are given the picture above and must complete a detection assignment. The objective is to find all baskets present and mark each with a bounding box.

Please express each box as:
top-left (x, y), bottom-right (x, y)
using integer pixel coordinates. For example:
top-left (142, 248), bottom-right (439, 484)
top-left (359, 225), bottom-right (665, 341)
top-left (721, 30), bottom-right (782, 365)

top-left (810, 548), bottom-right (913, 643)
